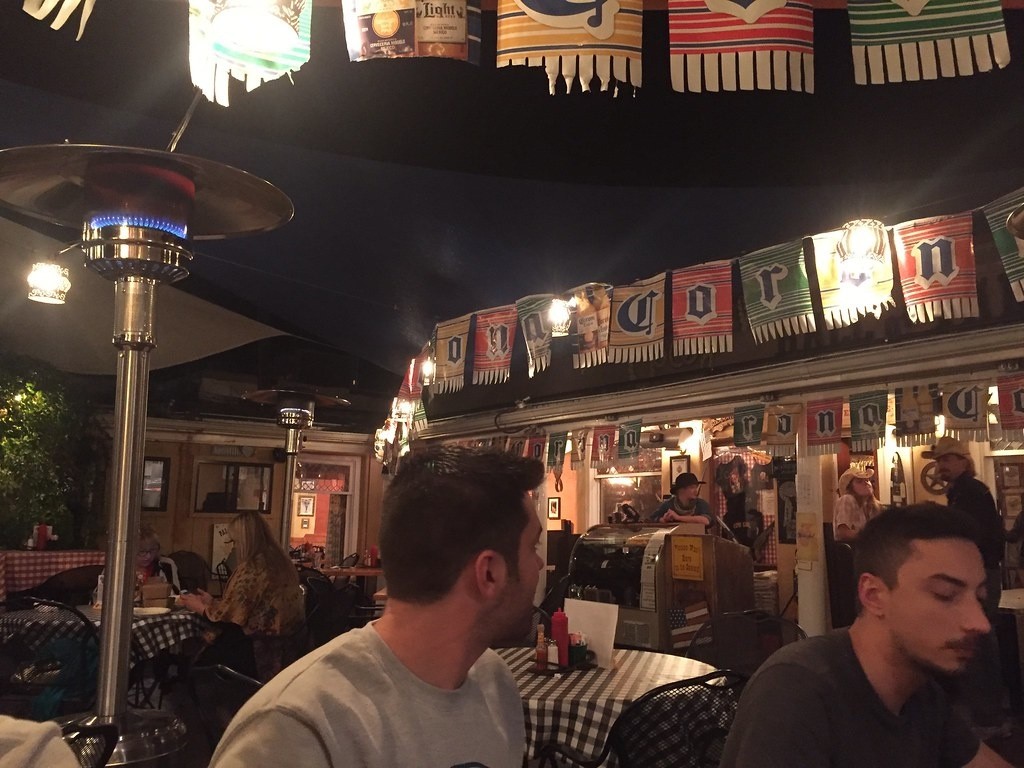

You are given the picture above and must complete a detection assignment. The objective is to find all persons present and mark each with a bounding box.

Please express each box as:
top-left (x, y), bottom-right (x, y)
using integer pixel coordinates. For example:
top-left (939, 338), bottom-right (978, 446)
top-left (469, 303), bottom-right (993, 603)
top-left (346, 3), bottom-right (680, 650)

top-left (832, 467), bottom-right (887, 541)
top-left (92, 521), bottom-right (180, 607)
top-left (934, 436), bottom-right (1013, 741)
top-left (650, 472), bottom-right (716, 534)
top-left (207, 445), bottom-right (545, 768)
top-left (720, 500), bottom-right (1016, 768)
top-left (176, 510), bottom-right (306, 666)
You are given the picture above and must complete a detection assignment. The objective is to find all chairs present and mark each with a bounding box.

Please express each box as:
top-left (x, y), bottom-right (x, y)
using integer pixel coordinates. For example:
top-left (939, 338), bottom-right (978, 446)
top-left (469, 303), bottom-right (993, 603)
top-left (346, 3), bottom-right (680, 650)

top-left (0, 544), bottom-right (810, 768)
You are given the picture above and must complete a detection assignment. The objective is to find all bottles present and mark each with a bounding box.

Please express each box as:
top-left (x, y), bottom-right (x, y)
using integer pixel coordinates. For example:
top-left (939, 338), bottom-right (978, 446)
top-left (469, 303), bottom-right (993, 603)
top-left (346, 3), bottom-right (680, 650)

top-left (535, 607), bottom-right (569, 670)
top-left (27, 521), bottom-right (59, 550)
top-left (364, 543), bottom-right (382, 567)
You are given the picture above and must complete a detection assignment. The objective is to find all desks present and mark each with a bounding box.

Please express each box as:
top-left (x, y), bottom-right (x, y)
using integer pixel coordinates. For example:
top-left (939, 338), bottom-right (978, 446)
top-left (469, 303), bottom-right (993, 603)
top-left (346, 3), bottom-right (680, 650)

top-left (490, 646), bottom-right (736, 768)
top-left (291, 564), bottom-right (383, 599)
top-left (288, 552), bottom-right (322, 572)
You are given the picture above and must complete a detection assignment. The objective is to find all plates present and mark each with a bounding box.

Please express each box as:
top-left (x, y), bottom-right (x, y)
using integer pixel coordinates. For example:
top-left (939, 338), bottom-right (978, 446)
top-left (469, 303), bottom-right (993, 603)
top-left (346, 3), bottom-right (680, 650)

top-left (169, 595), bottom-right (186, 610)
top-left (133, 608), bottom-right (171, 618)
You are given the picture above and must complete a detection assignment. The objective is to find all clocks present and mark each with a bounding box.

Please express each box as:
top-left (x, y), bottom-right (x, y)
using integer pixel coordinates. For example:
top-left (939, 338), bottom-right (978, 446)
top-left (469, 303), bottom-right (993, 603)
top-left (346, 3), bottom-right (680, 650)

top-left (241, 446), bottom-right (255, 457)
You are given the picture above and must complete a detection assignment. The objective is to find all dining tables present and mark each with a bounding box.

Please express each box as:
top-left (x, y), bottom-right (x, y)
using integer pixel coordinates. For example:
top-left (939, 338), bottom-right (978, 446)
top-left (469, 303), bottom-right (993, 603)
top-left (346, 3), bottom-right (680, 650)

top-left (1, 602), bottom-right (206, 709)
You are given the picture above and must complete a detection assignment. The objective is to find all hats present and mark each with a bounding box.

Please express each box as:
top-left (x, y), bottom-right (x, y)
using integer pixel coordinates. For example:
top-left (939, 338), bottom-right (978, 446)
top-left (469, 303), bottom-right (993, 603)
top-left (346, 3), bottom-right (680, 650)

top-left (838, 466), bottom-right (874, 496)
top-left (670, 473), bottom-right (707, 495)
top-left (923, 440), bottom-right (973, 463)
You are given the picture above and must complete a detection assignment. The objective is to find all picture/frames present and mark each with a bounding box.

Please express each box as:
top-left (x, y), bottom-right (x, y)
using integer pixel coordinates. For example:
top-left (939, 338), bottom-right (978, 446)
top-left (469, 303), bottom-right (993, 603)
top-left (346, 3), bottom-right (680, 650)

top-left (670, 455), bottom-right (690, 488)
top-left (298, 479), bottom-right (315, 529)
top-left (1000, 462), bottom-right (1024, 589)
top-left (547, 497), bottom-right (560, 520)
top-left (223, 465), bottom-right (247, 480)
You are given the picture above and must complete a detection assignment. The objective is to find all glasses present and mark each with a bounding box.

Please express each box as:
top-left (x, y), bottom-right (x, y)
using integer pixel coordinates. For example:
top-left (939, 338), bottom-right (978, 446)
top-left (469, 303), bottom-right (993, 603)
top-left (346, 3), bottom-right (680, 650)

top-left (136, 548), bottom-right (158, 556)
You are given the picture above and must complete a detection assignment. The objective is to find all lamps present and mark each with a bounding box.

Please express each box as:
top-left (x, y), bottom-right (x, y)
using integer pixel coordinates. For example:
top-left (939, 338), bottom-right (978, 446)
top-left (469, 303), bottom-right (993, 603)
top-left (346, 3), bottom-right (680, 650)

top-left (547, 290), bottom-right (571, 326)
top-left (640, 422), bottom-right (693, 449)
top-left (379, 341), bottom-right (434, 441)
top-left (210, 0), bottom-right (307, 49)
top-left (837, 215), bottom-right (887, 288)
top-left (26, 239), bottom-right (84, 305)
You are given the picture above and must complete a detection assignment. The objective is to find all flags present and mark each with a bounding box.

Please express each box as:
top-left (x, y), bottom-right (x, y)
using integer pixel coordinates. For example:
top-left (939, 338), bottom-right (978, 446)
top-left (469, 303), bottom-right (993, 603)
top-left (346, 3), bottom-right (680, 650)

top-left (667, 601), bottom-right (713, 649)
